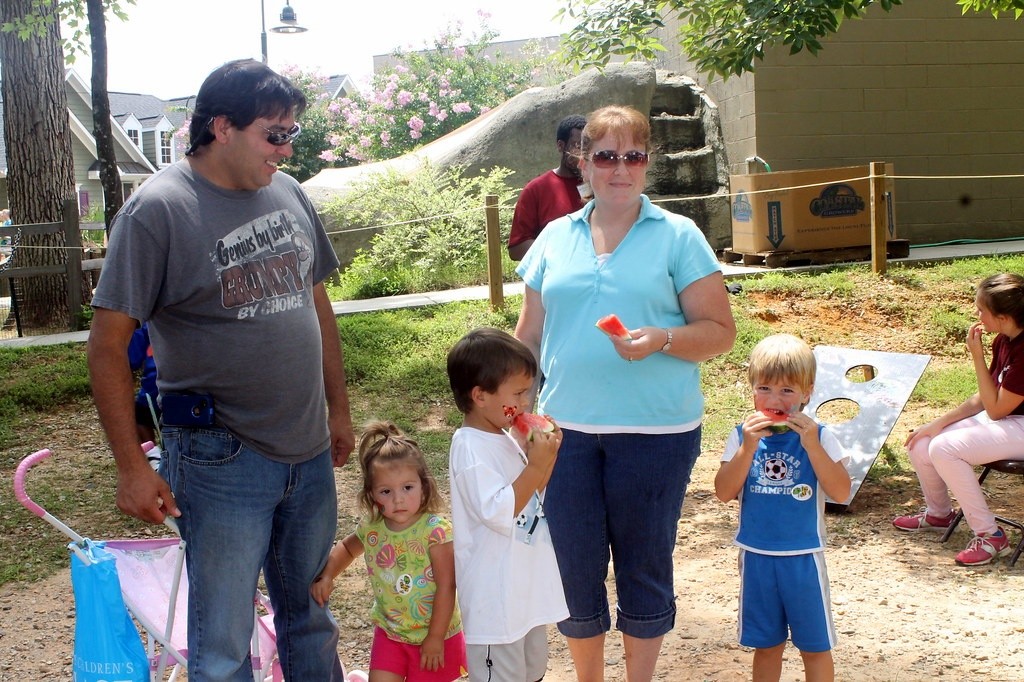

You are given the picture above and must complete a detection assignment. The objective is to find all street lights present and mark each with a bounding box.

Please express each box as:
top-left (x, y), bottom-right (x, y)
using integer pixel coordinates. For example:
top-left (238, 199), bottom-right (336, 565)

top-left (261, 0), bottom-right (309, 67)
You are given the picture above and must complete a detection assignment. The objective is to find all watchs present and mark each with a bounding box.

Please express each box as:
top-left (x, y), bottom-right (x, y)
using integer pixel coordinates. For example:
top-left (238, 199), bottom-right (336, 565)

top-left (659, 327), bottom-right (672, 353)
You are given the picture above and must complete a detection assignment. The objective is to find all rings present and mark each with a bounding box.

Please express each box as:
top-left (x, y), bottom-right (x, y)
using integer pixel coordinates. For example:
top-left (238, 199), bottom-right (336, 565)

top-left (629, 356), bottom-right (632, 361)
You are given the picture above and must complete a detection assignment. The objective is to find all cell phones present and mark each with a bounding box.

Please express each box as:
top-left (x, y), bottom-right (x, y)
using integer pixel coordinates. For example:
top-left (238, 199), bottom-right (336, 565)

top-left (162, 394), bottom-right (214, 425)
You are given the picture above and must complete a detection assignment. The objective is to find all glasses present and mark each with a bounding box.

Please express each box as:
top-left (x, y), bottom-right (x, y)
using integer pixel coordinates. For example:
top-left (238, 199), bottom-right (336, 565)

top-left (582, 149), bottom-right (650, 169)
top-left (206, 116), bottom-right (301, 146)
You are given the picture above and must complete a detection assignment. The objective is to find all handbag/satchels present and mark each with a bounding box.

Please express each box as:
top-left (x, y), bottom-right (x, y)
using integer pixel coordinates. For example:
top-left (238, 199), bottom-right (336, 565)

top-left (70, 538), bottom-right (152, 682)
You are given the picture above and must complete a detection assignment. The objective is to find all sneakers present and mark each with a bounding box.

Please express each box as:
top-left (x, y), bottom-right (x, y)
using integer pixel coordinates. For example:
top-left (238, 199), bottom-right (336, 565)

top-left (892, 507), bottom-right (961, 533)
top-left (954, 526), bottom-right (1013, 567)
top-left (144, 446), bottom-right (163, 472)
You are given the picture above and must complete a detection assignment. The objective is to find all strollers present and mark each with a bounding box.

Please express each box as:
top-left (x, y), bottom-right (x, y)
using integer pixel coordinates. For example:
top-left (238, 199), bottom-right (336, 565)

top-left (13, 441), bottom-right (369, 682)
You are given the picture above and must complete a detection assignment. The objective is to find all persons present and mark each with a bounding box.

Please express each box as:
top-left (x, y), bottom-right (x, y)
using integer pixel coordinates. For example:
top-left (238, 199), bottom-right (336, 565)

top-left (86, 58), bottom-right (354, 682)
top-left (509, 105), bottom-right (736, 682)
top-left (310, 417), bottom-right (468, 682)
top-left (893, 275), bottom-right (1024, 568)
top-left (0, 209), bottom-right (11, 262)
top-left (131, 322), bottom-right (160, 447)
top-left (714, 332), bottom-right (851, 682)
top-left (448, 326), bottom-right (572, 682)
top-left (507, 114), bottom-right (593, 261)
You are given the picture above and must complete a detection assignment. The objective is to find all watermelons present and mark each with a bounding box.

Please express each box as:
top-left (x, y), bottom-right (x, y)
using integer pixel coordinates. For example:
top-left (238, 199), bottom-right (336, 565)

top-left (514, 413), bottom-right (554, 442)
top-left (756, 410), bottom-right (795, 432)
top-left (596, 313), bottom-right (632, 342)
